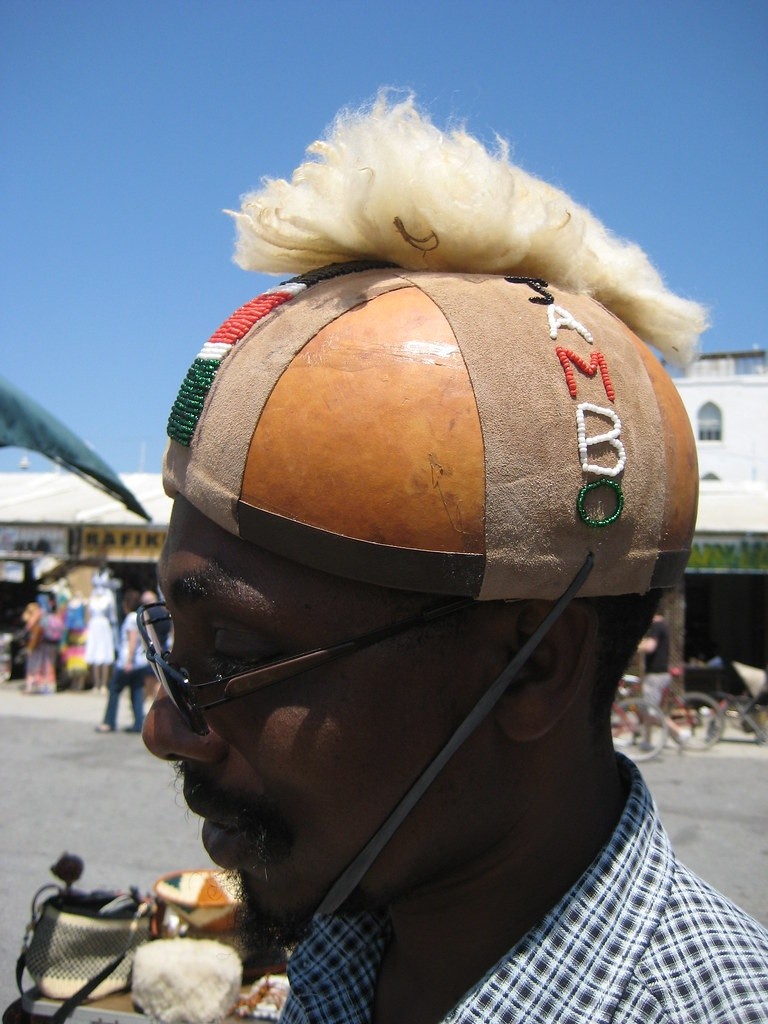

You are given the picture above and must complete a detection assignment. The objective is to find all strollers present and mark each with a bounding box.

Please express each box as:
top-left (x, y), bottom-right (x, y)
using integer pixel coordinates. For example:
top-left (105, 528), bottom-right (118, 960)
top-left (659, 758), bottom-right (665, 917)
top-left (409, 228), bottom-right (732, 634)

top-left (686, 657), bottom-right (768, 748)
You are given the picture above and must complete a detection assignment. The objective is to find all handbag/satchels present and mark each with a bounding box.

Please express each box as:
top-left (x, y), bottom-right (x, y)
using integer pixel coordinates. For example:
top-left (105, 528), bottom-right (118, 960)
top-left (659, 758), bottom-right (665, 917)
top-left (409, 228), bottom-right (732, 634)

top-left (16, 882), bottom-right (154, 1024)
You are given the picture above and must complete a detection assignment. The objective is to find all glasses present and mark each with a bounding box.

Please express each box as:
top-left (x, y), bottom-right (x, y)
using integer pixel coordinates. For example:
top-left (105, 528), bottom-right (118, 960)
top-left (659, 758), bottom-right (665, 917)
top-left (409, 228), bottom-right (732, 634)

top-left (138, 586), bottom-right (489, 712)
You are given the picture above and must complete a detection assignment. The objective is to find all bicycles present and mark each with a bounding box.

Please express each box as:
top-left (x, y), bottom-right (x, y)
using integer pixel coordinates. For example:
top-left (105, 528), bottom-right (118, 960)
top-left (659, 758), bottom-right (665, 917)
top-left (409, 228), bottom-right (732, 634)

top-left (612, 668), bottom-right (725, 762)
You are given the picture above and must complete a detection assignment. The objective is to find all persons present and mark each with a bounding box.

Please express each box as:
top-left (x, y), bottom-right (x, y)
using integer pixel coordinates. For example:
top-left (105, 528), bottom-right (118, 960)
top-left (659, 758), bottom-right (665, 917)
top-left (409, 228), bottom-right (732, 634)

top-left (24, 594), bottom-right (57, 694)
top-left (136, 84), bottom-right (768, 1024)
top-left (37, 556), bottom-right (123, 694)
top-left (637, 604), bottom-right (692, 751)
top-left (94, 590), bottom-right (148, 733)
top-left (140, 590), bottom-right (171, 703)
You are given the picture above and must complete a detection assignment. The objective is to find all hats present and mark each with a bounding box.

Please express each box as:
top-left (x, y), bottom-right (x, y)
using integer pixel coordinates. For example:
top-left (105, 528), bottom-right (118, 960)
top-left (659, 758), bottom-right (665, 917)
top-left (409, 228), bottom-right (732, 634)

top-left (163, 100), bottom-right (713, 603)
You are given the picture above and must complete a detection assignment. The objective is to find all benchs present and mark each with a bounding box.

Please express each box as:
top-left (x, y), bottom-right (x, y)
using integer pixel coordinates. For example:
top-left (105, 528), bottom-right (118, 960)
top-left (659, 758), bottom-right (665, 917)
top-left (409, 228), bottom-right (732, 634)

top-left (21, 987), bottom-right (271, 1023)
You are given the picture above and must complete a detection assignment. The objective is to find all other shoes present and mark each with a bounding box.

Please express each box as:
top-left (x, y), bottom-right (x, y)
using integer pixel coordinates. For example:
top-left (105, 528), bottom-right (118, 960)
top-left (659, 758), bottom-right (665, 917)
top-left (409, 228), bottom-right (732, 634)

top-left (95, 723), bottom-right (115, 732)
top-left (123, 726), bottom-right (141, 733)
top-left (676, 728), bottom-right (691, 755)
top-left (638, 741), bottom-right (652, 750)
